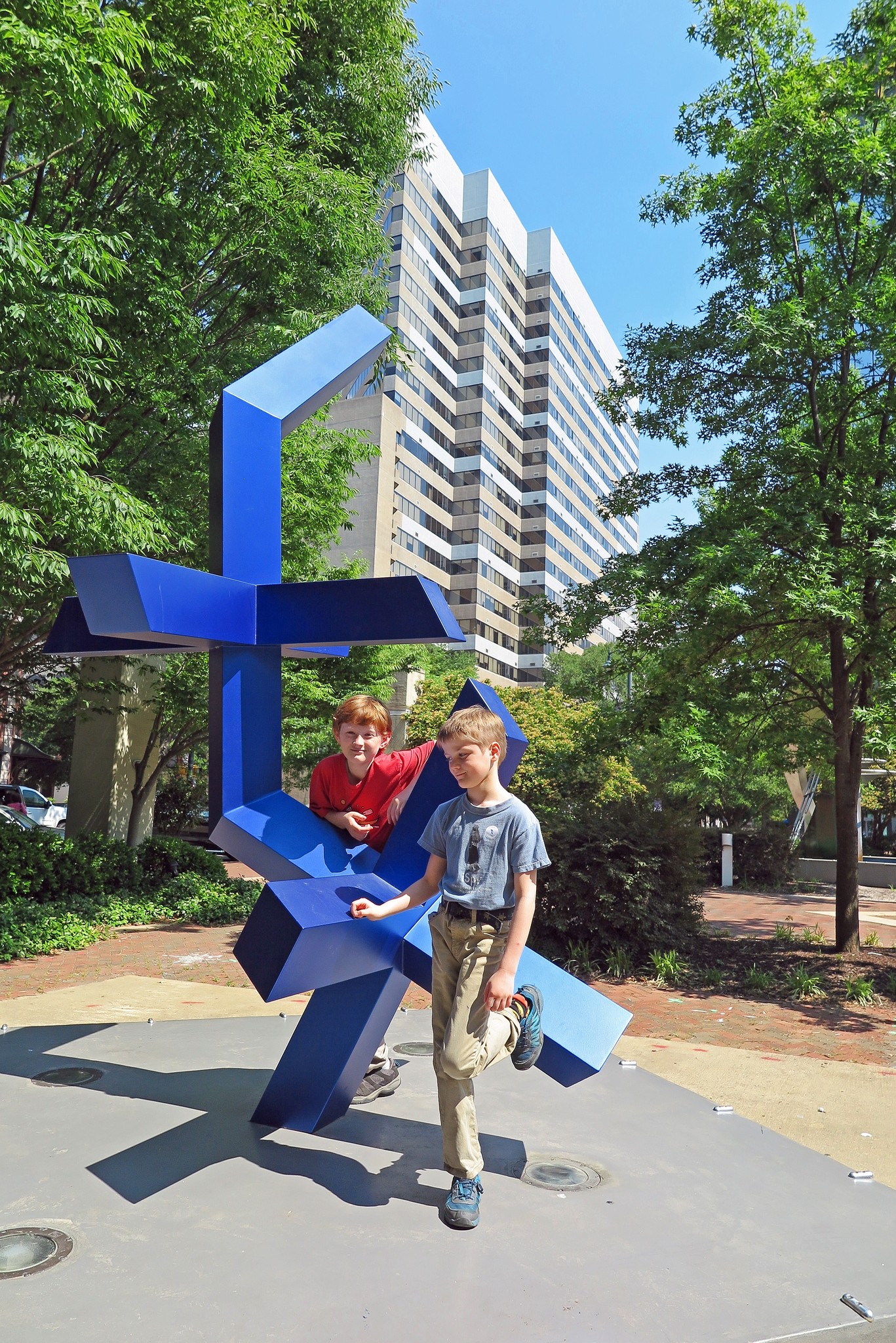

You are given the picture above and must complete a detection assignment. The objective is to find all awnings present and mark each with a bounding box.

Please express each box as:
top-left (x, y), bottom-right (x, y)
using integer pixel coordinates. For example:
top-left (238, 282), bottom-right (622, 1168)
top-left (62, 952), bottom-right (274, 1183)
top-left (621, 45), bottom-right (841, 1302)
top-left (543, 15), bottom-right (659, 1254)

top-left (11, 737), bottom-right (63, 784)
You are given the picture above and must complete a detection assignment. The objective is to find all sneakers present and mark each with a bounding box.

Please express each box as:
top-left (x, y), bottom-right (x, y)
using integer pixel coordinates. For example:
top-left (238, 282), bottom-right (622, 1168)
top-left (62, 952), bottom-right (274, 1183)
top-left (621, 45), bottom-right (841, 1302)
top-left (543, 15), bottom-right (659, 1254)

top-left (350, 1057), bottom-right (401, 1104)
top-left (444, 1173), bottom-right (484, 1228)
top-left (510, 984), bottom-right (543, 1071)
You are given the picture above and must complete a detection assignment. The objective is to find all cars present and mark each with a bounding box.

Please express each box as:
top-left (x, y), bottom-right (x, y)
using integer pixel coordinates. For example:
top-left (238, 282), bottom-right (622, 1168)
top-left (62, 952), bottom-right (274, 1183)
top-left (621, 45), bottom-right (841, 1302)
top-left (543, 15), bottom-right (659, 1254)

top-left (0, 804), bottom-right (66, 841)
top-left (0, 784), bottom-right (67, 829)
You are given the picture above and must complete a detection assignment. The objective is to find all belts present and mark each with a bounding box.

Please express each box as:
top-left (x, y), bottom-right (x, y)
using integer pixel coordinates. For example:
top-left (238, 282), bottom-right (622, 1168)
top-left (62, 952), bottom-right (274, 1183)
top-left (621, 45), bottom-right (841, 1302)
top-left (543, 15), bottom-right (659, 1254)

top-left (441, 896), bottom-right (512, 934)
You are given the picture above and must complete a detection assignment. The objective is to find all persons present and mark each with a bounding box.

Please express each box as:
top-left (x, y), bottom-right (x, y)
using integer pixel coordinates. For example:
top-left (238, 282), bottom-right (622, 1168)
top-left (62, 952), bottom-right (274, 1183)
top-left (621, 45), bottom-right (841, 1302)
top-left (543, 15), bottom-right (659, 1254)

top-left (350, 703), bottom-right (552, 1229)
top-left (310, 695), bottom-right (439, 1104)
top-left (7, 792), bottom-right (27, 814)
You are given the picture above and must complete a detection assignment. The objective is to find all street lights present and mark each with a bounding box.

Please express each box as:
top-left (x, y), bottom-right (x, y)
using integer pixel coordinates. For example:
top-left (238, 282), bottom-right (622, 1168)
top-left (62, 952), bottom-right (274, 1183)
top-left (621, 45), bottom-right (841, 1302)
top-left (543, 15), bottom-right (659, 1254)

top-left (603, 649), bottom-right (632, 713)
